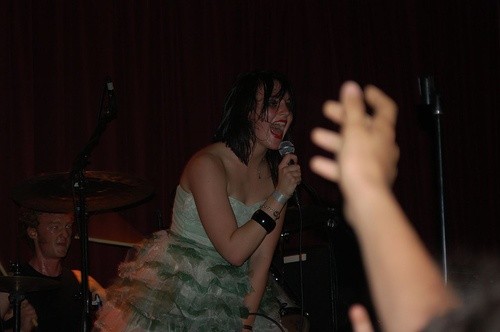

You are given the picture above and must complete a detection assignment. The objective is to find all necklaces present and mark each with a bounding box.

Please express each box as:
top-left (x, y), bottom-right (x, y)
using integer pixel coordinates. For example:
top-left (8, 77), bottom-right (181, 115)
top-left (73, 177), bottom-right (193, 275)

top-left (249, 159), bottom-right (266, 179)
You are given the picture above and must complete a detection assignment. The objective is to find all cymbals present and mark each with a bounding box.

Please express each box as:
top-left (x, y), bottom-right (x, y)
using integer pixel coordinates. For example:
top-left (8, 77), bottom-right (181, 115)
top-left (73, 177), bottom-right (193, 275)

top-left (0, 273), bottom-right (61, 294)
top-left (281, 206), bottom-right (339, 232)
top-left (10, 170), bottom-right (159, 213)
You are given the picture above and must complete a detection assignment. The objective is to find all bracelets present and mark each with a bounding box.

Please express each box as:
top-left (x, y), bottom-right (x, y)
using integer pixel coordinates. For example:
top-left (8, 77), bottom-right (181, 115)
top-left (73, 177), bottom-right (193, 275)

top-left (243, 324), bottom-right (252, 331)
top-left (271, 191), bottom-right (289, 205)
top-left (259, 204), bottom-right (279, 218)
top-left (252, 209), bottom-right (276, 234)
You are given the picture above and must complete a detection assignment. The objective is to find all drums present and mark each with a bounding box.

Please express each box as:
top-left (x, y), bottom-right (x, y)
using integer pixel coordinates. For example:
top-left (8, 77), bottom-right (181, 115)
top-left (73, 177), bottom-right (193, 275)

top-left (253, 262), bottom-right (312, 332)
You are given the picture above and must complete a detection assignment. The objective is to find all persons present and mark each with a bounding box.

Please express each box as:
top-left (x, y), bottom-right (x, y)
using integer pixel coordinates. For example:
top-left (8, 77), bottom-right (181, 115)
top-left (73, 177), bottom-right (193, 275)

top-left (119, 69), bottom-right (301, 332)
top-left (309, 80), bottom-right (500, 332)
top-left (0, 206), bottom-right (109, 332)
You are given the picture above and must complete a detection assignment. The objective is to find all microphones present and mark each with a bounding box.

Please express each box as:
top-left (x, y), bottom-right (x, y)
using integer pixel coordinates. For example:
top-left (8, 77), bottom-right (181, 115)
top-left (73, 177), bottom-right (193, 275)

top-left (279, 140), bottom-right (295, 167)
